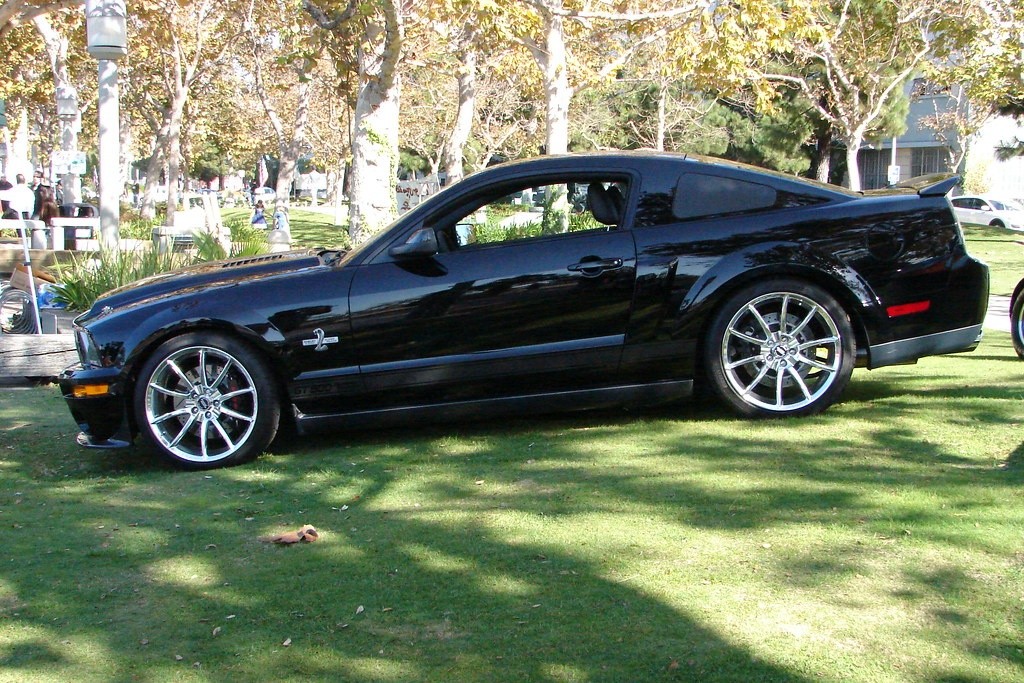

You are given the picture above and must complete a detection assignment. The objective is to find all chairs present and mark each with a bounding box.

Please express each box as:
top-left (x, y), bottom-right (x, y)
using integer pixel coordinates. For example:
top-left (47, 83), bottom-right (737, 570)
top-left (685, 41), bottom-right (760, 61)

top-left (584, 181), bottom-right (625, 232)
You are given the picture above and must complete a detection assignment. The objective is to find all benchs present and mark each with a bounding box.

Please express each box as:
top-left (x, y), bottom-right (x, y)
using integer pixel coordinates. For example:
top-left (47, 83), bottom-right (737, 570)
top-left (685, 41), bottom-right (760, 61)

top-left (152, 226), bottom-right (232, 259)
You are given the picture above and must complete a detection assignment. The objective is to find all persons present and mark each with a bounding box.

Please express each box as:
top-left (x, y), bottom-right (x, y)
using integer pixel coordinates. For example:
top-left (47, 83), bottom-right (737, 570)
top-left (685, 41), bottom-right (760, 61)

top-left (572, 187), bottom-right (583, 205)
top-left (251, 200), bottom-right (273, 224)
top-left (0, 171), bottom-right (64, 238)
top-left (274, 206), bottom-right (289, 230)
top-left (250, 179), bottom-right (258, 206)
top-left (84, 207), bottom-right (93, 217)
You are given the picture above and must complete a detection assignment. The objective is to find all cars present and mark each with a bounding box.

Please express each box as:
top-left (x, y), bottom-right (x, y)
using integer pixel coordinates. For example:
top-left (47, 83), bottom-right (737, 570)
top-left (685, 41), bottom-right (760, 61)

top-left (144, 183), bottom-right (275, 206)
top-left (57, 151), bottom-right (989, 467)
top-left (950, 194), bottom-right (1024, 231)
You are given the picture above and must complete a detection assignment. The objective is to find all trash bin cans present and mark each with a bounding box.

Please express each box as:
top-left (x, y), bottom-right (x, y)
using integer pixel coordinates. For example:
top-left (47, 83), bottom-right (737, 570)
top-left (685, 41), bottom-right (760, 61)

top-left (60, 203), bottom-right (98, 251)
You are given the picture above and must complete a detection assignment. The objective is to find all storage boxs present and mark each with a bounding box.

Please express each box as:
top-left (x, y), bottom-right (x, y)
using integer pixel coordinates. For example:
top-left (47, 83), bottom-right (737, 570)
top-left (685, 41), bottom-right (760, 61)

top-left (8, 263), bottom-right (58, 298)
